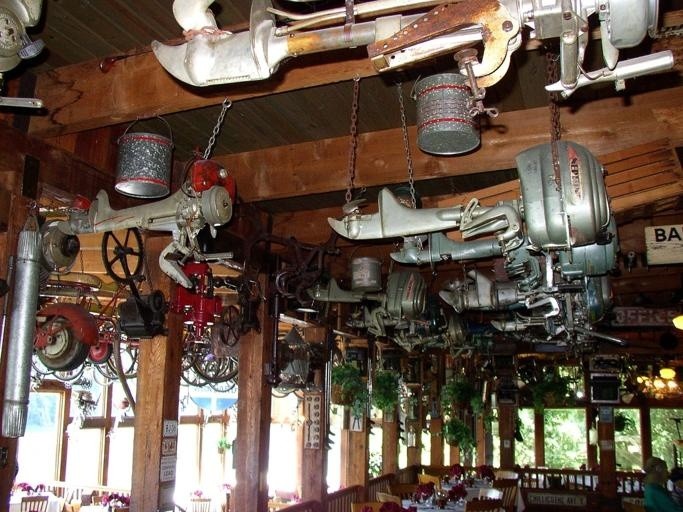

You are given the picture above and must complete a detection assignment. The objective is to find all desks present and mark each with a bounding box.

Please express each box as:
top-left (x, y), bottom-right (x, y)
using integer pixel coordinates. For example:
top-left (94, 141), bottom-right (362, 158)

top-left (9, 491), bottom-right (64, 511)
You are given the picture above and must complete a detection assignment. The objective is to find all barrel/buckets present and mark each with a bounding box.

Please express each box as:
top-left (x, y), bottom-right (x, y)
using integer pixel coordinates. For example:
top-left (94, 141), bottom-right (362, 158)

top-left (349, 246), bottom-right (383, 293)
top-left (114, 116), bottom-right (174, 200)
top-left (408, 72), bottom-right (483, 157)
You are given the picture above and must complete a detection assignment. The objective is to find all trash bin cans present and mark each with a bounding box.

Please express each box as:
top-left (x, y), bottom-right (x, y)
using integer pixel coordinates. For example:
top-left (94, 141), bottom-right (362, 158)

top-left (547, 476), bottom-right (562, 490)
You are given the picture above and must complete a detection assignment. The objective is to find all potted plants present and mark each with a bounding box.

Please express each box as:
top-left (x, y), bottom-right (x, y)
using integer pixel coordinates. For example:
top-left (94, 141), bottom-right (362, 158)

top-left (329, 362), bottom-right (369, 419)
top-left (440, 417), bottom-right (474, 453)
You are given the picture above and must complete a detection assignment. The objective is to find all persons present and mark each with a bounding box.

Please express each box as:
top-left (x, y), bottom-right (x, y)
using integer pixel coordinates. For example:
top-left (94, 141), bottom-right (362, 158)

top-left (573, 464), bottom-right (587, 482)
top-left (643, 457), bottom-right (682, 512)
top-left (668, 467), bottom-right (682, 506)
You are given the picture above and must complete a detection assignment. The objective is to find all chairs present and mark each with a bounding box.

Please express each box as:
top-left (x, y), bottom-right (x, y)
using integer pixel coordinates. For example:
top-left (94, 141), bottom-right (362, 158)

top-left (19, 480), bottom-right (131, 511)
top-left (348, 472), bottom-right (518, 512)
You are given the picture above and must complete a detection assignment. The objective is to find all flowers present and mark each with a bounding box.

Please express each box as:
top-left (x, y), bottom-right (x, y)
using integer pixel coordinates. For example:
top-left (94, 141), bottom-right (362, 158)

top-left (450, 464), bottom-right (495, 481)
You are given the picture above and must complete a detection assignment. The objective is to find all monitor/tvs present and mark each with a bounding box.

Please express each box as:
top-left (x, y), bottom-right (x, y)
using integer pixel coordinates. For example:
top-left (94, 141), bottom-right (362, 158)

top-left (590, 371), bottom-right (621, 404)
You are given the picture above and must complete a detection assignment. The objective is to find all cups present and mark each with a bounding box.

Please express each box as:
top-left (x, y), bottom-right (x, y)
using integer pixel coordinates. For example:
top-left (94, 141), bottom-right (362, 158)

top-left (71, 499), bottom-right (80, 512)
